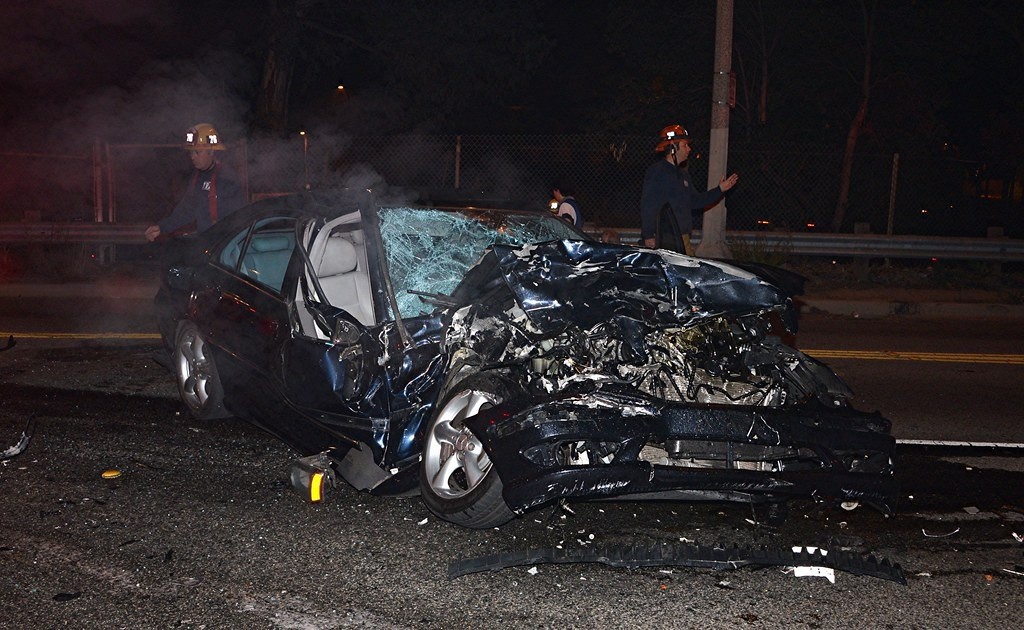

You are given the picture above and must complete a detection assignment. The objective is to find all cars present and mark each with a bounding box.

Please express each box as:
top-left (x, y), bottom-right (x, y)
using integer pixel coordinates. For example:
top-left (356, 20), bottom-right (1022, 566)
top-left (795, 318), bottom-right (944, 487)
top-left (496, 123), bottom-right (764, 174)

top-left (152, 192), bottom-right (900, 527)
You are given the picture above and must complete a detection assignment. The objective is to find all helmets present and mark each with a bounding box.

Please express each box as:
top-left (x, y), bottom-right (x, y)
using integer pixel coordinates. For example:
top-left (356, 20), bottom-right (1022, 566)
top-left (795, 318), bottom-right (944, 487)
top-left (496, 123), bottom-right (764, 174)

top-left (180, 123), bottom-right (226, 150)
top-left (655, 125), bottom-right (692, 152)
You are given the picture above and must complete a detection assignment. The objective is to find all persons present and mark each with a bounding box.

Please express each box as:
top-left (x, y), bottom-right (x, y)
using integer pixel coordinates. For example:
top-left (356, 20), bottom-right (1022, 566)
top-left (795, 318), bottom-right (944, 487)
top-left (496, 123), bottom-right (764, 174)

top-left (637, 124), bottom-right (739, 257)
top-left (142, 120), bottom-right (244, 373)
top-left (545, 178), bottom-right (583, 232)
